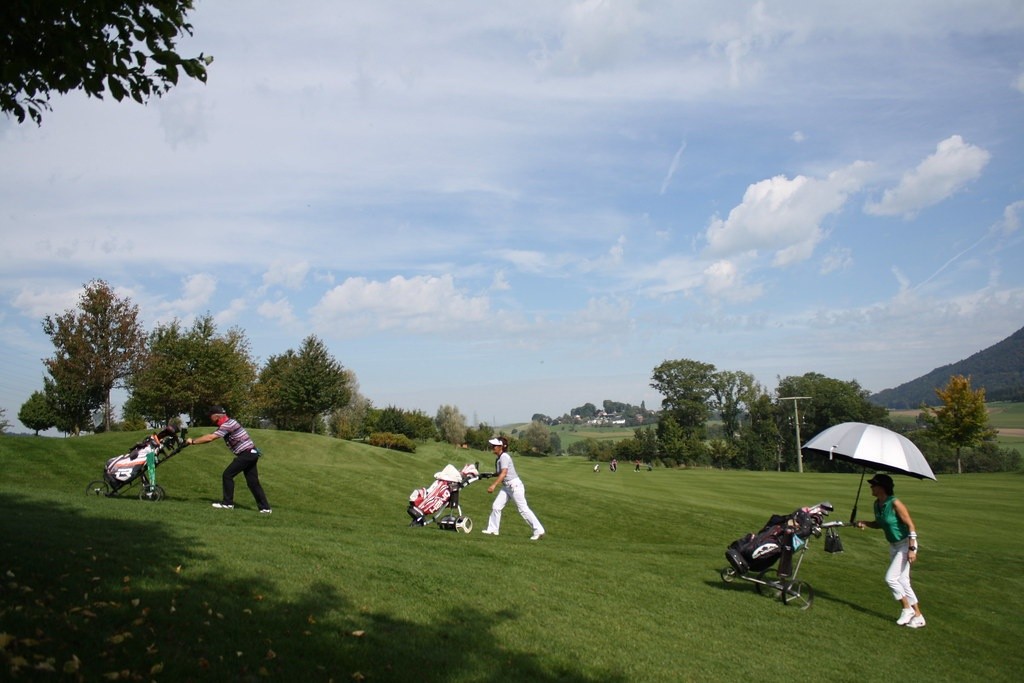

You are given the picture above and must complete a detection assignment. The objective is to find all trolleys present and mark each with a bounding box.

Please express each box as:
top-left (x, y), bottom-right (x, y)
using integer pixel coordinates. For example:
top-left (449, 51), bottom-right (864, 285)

top-left (592, 464), bottom-right (601, 473)
top-left (86, 424), bottom-right (191, 502)
top-left (720, 500), bottom-right (858, 610)
top-left (407, 460), bottom-right (493, 534)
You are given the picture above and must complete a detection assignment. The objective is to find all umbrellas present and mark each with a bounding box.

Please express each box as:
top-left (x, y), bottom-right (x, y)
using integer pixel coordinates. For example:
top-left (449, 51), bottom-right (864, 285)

top-left (800, 421), bottom-right (938, 523)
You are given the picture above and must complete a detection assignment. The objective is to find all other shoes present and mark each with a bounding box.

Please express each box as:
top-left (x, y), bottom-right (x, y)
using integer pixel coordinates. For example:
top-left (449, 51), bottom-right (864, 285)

top-left (212, 502), bottom-right (235, 509)
top-left (260, 507), bottom-right (272, 514)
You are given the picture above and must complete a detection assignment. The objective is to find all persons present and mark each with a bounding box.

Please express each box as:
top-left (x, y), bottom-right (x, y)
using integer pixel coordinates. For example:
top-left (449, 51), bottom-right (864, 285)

top-left (480, 436), bottom-right (546, 539)
top-left (855, 474), bottom-right (926, 628)
top-left (186, 407), bottom-right (271, 512)
top-left (612, 457), bottom-right (651, 472)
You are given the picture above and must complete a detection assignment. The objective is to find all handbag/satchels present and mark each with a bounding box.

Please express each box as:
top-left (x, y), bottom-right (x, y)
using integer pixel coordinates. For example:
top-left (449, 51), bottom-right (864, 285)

top-left (824, 525), bottom-right (844, 553)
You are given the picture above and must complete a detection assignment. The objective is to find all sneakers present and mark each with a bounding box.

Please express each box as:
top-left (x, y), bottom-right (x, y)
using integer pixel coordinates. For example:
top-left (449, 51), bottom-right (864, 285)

top-left (896, 604), bottom-right (916, 625)
top-left (905, 615), bottom-right (927, 629)
top-left (529, 531), bottom-right (545, 541)
top-left (482, 529), bottom-right (499, 536)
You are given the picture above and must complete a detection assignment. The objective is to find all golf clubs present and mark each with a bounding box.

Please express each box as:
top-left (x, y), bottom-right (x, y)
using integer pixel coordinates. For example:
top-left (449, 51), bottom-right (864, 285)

top-left (808, 501), bottom-right (834, 517)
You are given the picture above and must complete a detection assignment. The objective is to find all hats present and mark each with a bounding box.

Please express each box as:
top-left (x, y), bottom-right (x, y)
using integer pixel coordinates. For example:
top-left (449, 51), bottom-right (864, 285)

top-left (210, 405), bottom-right (226, 415)
top-left (490, 438), bottom-right (504, 446)
top-left (867, 473), bottom-right (895, 491)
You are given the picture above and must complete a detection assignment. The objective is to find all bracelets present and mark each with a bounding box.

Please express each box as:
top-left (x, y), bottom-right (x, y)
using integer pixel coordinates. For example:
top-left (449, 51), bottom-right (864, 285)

top-left (908, 546), bottom-right (915, 550)
top-left (191, 439), bottom-right (195, 445)
